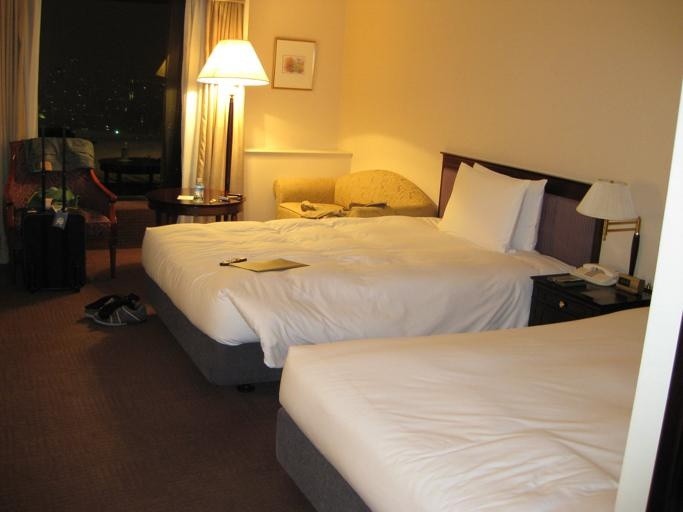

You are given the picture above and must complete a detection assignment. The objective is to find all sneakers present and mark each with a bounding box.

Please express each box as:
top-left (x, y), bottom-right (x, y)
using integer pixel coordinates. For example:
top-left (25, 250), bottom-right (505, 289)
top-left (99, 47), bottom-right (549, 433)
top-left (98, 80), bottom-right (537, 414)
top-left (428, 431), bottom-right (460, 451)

top-left (84, 292), bottom-right (148, 327)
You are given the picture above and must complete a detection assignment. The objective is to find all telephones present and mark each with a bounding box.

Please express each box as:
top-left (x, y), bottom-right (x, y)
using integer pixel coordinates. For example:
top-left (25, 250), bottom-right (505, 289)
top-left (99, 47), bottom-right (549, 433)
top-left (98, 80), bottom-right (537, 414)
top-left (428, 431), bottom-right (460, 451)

top-left (570, 263), bottom-right (617, 286)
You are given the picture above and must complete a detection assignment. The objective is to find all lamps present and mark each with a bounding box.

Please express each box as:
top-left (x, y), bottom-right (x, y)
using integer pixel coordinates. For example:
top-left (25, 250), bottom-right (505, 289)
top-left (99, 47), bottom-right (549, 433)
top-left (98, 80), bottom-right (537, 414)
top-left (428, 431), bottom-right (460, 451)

top-left (197, 36), bottom-right (271, 196)
top-left (573, 177), bottom-right (644, 275)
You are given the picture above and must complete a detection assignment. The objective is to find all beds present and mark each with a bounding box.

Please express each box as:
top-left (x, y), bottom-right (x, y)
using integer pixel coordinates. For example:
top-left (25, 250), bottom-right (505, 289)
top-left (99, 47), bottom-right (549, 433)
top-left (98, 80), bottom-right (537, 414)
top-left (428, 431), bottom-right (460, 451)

top-left (272, 303), bottom-right (649, 512)
top-left (139, 153), bottom-right (605, 392)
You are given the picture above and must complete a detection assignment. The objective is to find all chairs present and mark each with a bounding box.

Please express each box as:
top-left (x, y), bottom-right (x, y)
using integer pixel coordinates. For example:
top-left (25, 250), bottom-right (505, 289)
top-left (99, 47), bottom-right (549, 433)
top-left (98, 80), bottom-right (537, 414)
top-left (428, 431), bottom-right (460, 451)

top-left (4, 137), bottom-right (118, 278)
top-left (271, 169), bottom-right (438, 217)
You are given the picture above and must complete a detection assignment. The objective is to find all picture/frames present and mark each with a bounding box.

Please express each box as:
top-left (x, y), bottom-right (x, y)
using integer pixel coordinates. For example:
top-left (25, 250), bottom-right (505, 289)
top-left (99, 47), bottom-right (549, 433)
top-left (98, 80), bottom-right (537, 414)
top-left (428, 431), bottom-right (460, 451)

top-left (272, 37), bottom-right (318, 95)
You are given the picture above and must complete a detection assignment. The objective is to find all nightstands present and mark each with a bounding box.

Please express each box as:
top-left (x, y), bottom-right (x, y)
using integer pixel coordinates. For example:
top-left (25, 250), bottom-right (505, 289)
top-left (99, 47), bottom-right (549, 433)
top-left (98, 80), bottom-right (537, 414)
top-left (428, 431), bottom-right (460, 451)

top-left (527, 273), bottom-right (652, 327)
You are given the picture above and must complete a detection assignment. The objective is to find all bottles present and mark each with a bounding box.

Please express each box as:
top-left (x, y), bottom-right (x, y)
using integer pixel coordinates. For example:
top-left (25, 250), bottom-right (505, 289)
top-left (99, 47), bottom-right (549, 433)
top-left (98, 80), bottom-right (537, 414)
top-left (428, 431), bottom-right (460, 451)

top-left (194, 178), bottom-right (204, 203)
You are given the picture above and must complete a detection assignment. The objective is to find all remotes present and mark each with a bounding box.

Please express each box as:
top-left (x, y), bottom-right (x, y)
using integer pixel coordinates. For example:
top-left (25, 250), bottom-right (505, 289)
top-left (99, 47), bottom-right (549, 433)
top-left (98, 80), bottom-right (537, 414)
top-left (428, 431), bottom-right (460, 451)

top-left (549, 273), bottom-right (583, 283)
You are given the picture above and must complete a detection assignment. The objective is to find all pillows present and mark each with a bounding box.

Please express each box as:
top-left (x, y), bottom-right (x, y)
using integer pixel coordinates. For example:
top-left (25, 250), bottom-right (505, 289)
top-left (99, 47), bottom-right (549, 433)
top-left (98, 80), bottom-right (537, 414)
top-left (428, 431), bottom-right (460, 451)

top-left (469, 160), bottom-right (549, 253)
top-left (440, 161), bottom-right (532, 251)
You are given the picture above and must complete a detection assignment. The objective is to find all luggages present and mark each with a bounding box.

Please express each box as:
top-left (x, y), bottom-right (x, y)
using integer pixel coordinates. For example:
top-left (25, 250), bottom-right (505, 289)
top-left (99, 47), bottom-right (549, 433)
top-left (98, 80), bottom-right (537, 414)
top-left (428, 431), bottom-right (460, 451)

top-left (21, 208), bottom-right (87, 294)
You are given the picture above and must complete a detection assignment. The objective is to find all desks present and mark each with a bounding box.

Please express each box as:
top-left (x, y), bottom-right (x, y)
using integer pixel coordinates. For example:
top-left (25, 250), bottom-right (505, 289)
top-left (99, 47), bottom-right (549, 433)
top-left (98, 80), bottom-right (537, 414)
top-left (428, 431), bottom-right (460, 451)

top-left (149, 186), bottom-right (245, 221)
